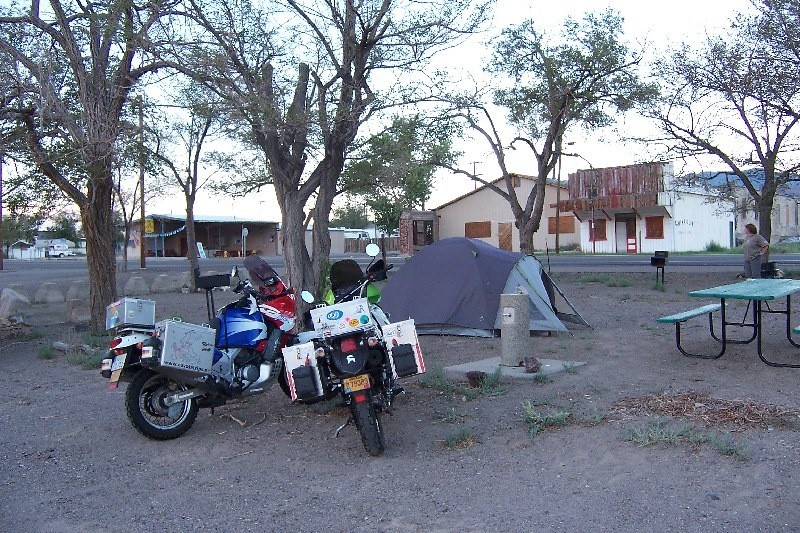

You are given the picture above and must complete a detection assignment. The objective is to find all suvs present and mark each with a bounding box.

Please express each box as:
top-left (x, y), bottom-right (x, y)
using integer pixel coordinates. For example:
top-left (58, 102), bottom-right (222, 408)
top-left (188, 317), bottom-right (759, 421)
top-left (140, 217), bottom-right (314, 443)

top-left (45, 244), bottom-right (69, 258)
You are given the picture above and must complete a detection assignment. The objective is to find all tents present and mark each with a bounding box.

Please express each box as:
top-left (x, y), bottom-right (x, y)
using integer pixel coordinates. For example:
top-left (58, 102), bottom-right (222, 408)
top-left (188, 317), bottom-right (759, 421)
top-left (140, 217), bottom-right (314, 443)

top-left (380, 238), bottom-right (591, 337)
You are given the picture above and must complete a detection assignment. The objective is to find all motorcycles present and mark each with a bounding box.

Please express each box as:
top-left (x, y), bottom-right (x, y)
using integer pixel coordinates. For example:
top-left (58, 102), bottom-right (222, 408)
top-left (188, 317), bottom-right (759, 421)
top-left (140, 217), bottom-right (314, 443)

top-left (99, 253), bottom-right (328, 442)
top-left (279, 243), bottom-right (427, 456)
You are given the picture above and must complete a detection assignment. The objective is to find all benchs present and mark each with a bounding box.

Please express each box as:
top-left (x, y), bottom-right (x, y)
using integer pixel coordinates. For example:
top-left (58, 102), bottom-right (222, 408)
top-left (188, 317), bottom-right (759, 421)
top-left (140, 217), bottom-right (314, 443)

top-left (656, 302), bottom-right (800, 368)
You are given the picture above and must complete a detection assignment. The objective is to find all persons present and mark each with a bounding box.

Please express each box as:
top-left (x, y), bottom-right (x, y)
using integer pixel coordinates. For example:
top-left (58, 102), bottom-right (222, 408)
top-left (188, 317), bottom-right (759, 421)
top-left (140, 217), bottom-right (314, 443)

top-left (743, 223), bottom-right (770, 279)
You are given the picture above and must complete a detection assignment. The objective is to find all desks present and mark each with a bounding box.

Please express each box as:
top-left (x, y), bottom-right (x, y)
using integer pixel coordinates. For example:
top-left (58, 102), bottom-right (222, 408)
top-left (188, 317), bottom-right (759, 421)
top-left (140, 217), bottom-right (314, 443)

top-left (688, 279), bottom-right (800, 367)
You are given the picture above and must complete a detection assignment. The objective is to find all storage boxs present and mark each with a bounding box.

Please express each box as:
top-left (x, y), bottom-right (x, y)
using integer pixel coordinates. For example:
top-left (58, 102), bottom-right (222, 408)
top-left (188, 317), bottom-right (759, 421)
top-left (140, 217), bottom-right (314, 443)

top-left (159, 317), bottom-right (216, 374)
top-left (105, 297), bottom-right (156, 331)
top-left (280, 338), bottom-right (325, 403)
top-left (309, 296), bottom-right (372, 341)
top-left (381, 316), bottom-right (425, 380)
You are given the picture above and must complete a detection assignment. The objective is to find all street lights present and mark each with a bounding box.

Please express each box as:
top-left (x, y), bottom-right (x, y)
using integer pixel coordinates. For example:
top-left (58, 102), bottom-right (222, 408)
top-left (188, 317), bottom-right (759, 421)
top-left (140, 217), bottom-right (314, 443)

top-left (469, 160), bottom-right (483, 190)
top-left (556, 141), bottom-right (576, 254)
